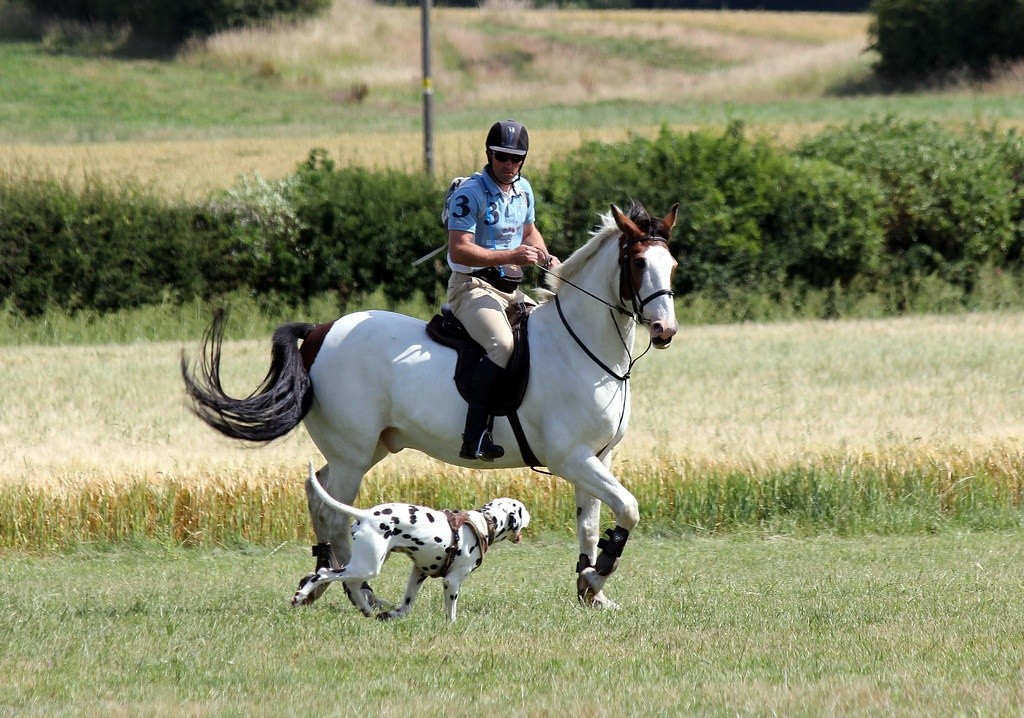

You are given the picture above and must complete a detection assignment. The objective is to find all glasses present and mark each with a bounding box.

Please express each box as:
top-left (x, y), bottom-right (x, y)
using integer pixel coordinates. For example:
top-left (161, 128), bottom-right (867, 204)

top-left (491, 150), bottom-right (524, 164)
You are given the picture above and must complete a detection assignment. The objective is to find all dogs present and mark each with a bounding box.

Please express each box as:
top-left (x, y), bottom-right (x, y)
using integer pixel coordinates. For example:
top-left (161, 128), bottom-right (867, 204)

top-left (291, 458), bottom-right (531, 622)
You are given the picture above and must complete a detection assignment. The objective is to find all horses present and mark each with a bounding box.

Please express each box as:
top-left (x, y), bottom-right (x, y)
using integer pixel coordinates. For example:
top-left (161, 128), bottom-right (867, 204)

top-left (179, 200), bottom-right (679, 612)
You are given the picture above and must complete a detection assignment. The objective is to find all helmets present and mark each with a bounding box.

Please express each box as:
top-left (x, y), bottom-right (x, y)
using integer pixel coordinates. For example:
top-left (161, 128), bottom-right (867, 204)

top-left (486, 120), bottom-right (529, 155)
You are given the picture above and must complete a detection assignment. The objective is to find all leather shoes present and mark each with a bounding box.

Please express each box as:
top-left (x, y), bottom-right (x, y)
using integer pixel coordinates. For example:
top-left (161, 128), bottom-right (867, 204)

top-left (463, 427), bottom-right (504, 458)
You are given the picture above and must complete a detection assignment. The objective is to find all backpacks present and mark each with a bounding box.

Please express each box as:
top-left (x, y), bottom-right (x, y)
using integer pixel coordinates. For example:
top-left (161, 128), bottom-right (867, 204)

top-left (441, 175), bottom-right (488, 226)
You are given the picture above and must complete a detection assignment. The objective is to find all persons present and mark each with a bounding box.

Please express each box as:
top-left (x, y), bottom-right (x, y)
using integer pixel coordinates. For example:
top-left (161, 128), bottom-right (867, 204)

top-left (446, 118), bottom-right (563, 460)
